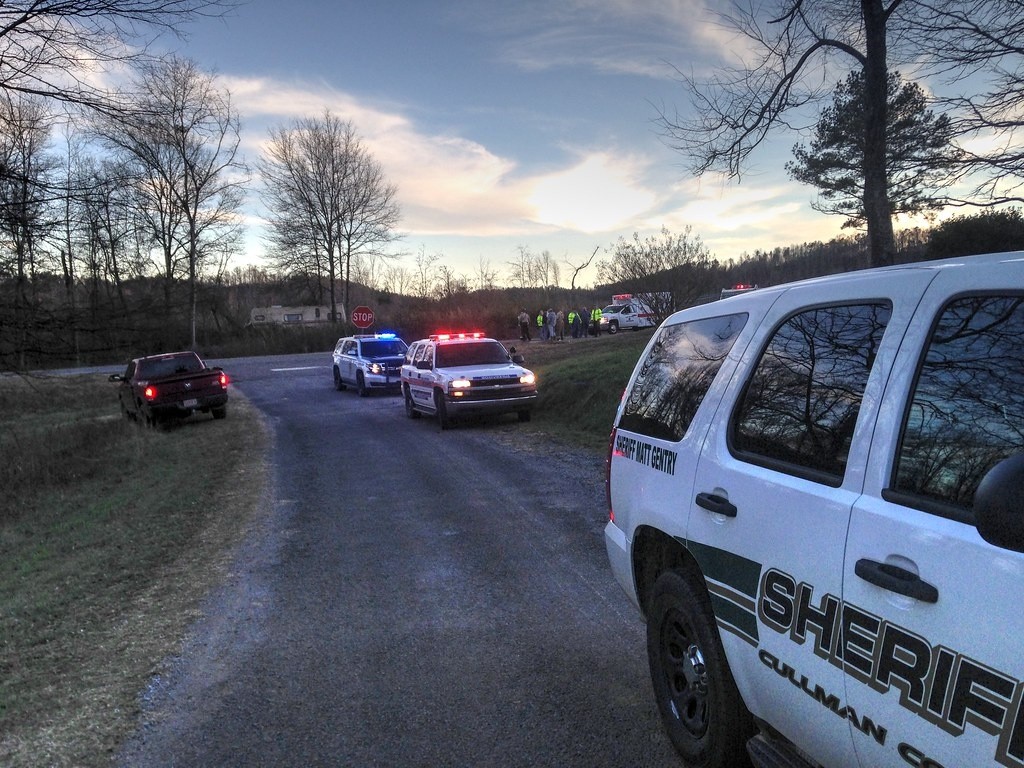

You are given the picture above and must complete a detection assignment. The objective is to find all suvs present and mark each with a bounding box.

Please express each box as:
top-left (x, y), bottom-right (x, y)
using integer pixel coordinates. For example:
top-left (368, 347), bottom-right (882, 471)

top-left (400, 333), bottom-right (538, 429)
top-left (602, 253), bottom-right (1024, 768)
top-left (334, 334), bottom-right (409, 397)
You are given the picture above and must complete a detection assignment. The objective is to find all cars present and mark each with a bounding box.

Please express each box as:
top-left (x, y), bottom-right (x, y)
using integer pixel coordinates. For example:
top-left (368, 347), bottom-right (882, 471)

top-left (720, 284), bottom-right (758, 301)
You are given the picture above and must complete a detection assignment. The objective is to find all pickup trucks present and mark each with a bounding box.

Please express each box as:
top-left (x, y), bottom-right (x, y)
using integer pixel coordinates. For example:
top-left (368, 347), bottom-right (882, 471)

top-left (108, 351), bottom-right (229, 433)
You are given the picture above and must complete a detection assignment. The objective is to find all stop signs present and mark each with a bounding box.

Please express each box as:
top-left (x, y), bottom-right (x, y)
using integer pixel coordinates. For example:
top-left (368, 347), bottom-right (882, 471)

top-left (350, 306), bottom-right (374, 329)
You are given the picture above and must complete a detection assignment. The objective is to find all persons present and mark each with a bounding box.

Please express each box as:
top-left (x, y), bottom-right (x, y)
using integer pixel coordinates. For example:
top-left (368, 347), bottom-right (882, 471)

top-left (517, 304), bottom-right (602, 343)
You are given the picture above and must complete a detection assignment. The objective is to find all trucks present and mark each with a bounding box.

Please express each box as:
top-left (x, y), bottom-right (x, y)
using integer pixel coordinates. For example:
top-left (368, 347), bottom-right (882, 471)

top-left (588, 292), bottom-right (677, 335)
